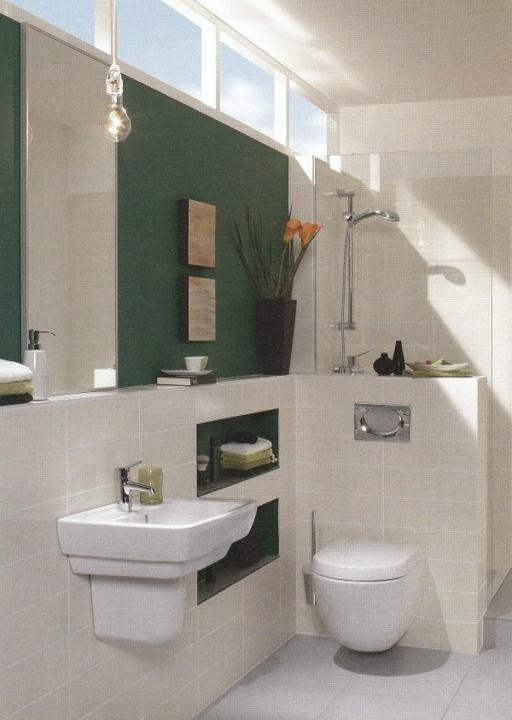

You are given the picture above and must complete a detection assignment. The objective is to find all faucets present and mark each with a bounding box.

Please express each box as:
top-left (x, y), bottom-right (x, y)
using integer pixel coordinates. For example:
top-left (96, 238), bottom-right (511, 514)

top-left (114, 460), bottom-right (155, 510)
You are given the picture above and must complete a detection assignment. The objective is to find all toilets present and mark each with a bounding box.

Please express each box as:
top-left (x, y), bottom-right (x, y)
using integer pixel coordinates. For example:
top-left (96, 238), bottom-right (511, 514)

top-left (312, 537), bottom-right (422, 653)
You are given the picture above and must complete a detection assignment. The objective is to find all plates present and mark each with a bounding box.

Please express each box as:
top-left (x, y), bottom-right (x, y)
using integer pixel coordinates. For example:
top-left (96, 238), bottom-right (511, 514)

top-left (161, 369), bottom-right (214, 375)
top-left (406, 362), bottom-right (469, 372)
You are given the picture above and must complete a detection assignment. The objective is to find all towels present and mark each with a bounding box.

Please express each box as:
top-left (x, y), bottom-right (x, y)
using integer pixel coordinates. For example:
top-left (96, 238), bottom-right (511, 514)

top-left (219, 433), bottom-right (278, 471)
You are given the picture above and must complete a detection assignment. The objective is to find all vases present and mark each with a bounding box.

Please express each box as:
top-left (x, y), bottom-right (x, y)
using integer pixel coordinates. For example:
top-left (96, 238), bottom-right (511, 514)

top-left (249, 294), bottom-right (300, 376)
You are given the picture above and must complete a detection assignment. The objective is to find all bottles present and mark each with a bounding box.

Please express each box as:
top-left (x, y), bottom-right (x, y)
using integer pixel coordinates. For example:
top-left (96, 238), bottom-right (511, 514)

top-left (373, 341), bottom-right (405, 375)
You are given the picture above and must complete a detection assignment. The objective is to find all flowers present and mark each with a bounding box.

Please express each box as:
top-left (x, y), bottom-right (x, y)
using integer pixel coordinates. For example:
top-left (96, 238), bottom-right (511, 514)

top-left (230, 204), bottom-right (325, 298)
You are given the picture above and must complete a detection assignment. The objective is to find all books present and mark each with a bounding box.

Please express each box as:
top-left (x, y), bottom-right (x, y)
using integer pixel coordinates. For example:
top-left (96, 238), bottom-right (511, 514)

top-left (156, 374), bottom-right (217, 387)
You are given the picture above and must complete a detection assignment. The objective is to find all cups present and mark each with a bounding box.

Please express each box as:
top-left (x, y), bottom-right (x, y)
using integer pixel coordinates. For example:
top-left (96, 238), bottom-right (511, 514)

top-left (184, 357), bottom-right (209, 371)
top-left (139, 467), bottom-right (164, 505)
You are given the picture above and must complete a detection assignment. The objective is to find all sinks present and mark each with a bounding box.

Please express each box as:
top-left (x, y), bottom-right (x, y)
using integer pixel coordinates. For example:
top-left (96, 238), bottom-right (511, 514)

top-left (57, 499), bottom-right (259, 581)
